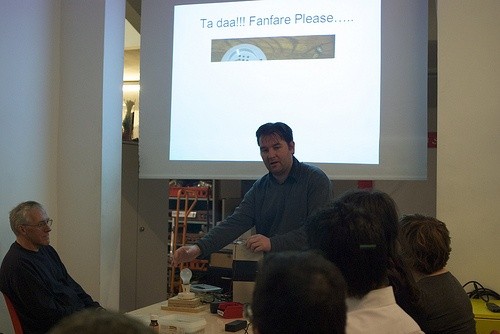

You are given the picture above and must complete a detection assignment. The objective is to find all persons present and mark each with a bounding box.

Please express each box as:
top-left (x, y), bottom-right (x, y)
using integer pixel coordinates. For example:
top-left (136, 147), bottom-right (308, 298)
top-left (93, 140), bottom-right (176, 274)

top-left (252, 252), bottom-right (347, 334)
top-left (173, 123), bottom-right (336, 264)
top-left (398, 214), bottom-right (477, 334)
top-left (0, 201), bottom-right (106, 334)
top-left (307, 188), bottom-right (423, 333)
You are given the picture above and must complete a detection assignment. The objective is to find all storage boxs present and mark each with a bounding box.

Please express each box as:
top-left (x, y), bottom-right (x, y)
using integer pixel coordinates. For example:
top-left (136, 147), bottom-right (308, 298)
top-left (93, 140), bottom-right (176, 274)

top-left (168, 182), bottom-right (211, 199)
top-left (470, 299), bottom-right (500, 334)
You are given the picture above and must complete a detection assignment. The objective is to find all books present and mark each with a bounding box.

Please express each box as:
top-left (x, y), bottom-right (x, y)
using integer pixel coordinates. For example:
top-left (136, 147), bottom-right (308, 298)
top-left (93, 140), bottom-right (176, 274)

top-left (161, 297), bottom-right (208, 313)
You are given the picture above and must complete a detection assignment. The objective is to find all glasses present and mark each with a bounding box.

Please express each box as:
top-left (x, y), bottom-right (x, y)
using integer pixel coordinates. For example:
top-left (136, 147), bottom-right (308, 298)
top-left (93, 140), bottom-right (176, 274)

top-left (22, 219), bottom-right (53, 228)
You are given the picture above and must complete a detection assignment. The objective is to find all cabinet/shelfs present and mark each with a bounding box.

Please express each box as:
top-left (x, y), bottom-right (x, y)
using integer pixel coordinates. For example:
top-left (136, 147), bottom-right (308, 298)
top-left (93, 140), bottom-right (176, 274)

top-left (167, 179), bottom-right (215, 285)
top-left (119, 141), bottom-right (168, 311)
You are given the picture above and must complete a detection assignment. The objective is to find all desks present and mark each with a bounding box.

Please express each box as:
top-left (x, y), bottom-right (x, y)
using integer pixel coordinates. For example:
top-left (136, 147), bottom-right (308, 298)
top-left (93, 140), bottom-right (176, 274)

top-left (126, 298), bottom-right (254, 334)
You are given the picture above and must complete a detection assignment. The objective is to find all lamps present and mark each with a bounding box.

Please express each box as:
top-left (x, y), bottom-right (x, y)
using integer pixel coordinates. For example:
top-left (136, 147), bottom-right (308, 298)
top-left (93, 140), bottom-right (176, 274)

top-left (178, 268), bottom-right (195, 300)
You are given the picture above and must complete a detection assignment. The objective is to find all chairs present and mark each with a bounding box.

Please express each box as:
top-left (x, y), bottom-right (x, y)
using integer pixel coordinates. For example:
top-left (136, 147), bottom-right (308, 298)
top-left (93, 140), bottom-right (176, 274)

top-left (2, 288), bottom-right (24, 334)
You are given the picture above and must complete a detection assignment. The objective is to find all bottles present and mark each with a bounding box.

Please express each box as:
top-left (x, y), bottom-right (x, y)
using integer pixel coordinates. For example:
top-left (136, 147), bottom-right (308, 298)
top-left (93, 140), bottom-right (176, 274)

top-left (148, 319), bottom-right (160, 334)
